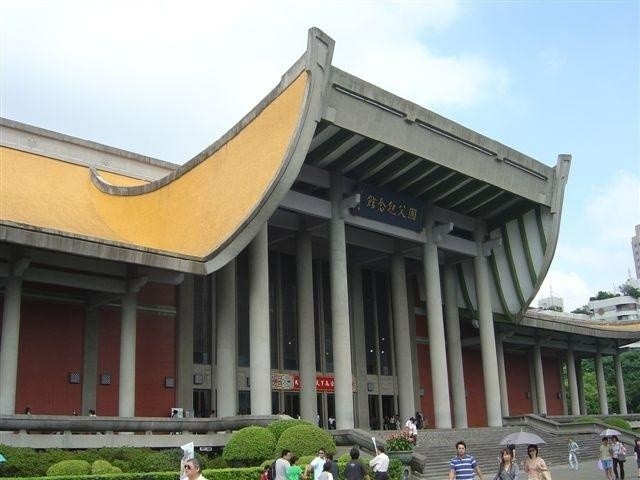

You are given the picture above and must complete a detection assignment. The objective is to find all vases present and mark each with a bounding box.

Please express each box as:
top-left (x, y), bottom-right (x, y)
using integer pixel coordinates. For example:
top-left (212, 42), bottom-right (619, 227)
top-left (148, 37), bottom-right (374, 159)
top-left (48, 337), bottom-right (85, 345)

top-left (384, 451), bottom-right (413, 480)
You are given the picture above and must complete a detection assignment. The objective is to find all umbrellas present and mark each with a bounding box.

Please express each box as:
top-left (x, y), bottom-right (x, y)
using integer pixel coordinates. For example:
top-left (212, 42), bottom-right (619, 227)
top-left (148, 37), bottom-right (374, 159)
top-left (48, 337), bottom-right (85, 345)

top-left (599, 428), bottom-right (622, 436)
top-left (497, 428), bottom-right (546, 445)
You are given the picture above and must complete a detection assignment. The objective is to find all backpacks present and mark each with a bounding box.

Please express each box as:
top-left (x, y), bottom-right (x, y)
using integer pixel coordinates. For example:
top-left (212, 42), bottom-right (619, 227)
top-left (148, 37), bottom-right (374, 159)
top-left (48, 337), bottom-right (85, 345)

top-left (267, 458), bottom-right (281, 479)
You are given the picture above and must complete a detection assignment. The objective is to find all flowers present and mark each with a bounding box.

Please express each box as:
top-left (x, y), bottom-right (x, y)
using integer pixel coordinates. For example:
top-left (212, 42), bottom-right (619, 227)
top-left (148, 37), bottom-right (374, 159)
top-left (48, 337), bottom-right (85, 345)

top-left (384, 427), bottom-right (414, 451)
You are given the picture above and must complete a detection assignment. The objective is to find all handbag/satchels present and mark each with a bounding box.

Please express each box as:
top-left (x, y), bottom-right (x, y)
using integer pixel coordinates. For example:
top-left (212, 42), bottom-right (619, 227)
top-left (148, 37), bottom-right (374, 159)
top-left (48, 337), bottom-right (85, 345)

top-left (574, 449), bottom-right (581, 456)
top-left (538, 471), bottom-right (552, 480)
top-left (597, 458), bottom-right (605, 471)
top-left (618, 454), bottom-right (626, 463)
top-left (494, 472), bottom-right (501, 480)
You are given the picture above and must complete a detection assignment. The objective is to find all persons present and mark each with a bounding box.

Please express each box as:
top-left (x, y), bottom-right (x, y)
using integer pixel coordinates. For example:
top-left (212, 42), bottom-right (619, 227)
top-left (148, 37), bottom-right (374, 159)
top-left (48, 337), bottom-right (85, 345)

top-left (492, 448), bottom-right (520, 480)
top-left (259, 410), bottom-right (424, 480)
top-left (25, 407), bottom-right (31, 415)
top-left (72, 408), bottom-right (97, 416)
top-left (448, 440), bottom-right (485, 480)
top-left (209, 410), bottom-right (216, 417)
top-left (610, 436), bottom-right (625, 480)
top-left (634, 438), bottom-right (639, 477)
top-left (568, 437), bottom-right (579, 471)
top-left (171, 410), bottom-right (179, 419)
top-left (507, 444), bottom-right (515, 461)
top-left (524, 445), bottom-right (548, 480)
top-left (182, 457), bottom-right (208, 480)
top-left (599, 438), bottom-right (615, 480)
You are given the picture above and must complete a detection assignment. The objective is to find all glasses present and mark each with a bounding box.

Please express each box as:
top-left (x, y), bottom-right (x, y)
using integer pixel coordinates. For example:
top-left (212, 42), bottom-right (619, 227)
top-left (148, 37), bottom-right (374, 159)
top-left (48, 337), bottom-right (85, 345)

top-left (183, 465), bottom-right (191, 470)
